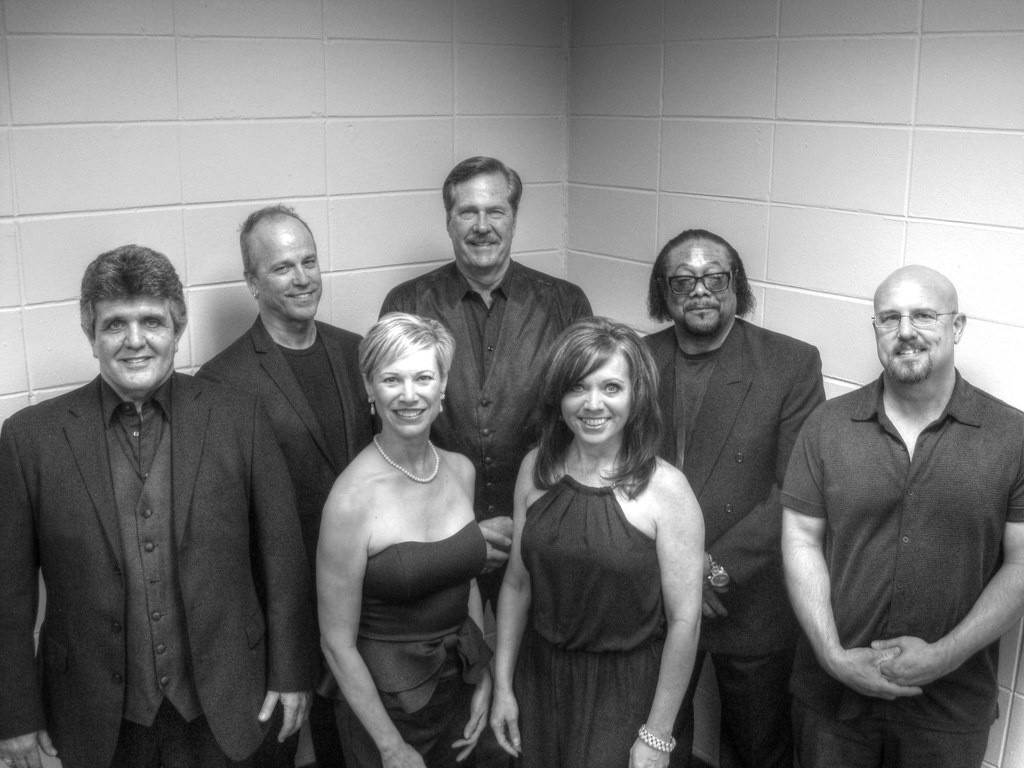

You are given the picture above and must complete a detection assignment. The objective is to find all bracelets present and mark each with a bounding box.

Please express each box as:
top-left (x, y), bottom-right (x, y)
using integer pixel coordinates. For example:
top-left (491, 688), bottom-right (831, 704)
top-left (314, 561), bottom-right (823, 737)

top-left (638, 726), bottom-right (675, 752)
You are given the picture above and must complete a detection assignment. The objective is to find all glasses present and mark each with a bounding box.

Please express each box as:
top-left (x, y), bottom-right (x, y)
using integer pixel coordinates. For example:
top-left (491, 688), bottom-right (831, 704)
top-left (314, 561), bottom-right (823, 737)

top-left (871, 310), bottom-right (957, 328)
top-left (663, 271), bottom-right (734, 296)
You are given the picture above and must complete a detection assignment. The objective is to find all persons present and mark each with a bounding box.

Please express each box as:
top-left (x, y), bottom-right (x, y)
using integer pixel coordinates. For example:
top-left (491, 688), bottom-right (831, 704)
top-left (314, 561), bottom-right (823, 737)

top-left (782, 264), bottom-right (1024, 768)
top-left (490, 315), bottom-right (704, 768)
top-left (318, 311), bottom-right (493, 768)
top-left (193, 204), bottom-right (382, 768)
top-left (640, 228), bottom-right (826, 768)
top-left (376, 155), bottom-right (592, 522)
top-left (1, 244), bottom-right (319, 768)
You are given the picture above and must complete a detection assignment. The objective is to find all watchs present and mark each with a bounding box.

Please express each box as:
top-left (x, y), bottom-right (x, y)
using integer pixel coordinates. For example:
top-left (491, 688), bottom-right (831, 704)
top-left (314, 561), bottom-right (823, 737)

top-left (708, 553), bottom-right (729, 587)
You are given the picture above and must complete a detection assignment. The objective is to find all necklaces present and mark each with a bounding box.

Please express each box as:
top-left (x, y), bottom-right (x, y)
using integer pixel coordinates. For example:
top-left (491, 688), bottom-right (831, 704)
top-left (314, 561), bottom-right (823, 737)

top-left (373, 433), bottom-right (440, 483)
